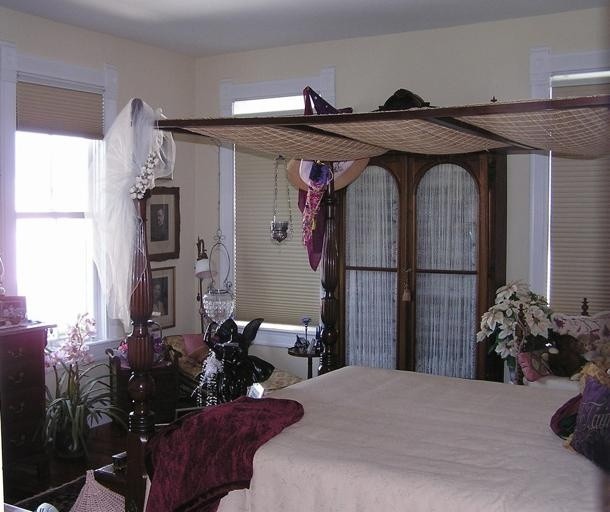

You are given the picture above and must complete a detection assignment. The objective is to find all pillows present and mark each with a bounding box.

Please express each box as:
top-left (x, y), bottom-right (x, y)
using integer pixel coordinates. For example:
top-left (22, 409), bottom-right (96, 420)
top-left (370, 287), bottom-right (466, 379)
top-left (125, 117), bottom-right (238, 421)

top-left (517, 352), bottom-right (552, 382)
top-left (563, 373), bottom-right (610, 476)
top-left (183, 334), bottom-right (203, 352)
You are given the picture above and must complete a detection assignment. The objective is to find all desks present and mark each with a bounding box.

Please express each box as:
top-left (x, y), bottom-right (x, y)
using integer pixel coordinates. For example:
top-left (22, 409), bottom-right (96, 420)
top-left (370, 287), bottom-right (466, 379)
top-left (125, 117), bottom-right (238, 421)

top-left (288, 346), bottom-right (320, 379)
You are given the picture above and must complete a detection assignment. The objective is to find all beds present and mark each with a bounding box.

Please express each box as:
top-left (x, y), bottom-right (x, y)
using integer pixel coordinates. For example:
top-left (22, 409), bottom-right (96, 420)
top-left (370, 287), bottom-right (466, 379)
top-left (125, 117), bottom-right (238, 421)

top-left (93, 98), bottom-right (610, 512)
top-left (163, 334), bottom-right (305, 405)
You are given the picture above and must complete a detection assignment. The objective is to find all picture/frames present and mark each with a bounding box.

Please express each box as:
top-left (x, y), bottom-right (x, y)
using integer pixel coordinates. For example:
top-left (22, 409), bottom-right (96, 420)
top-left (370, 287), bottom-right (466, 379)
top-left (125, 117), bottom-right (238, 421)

top-left (140, 186), bottom-right (181, 262)
top-left (150, 266), bottom-right (177, 331)
top-left (0, 296), bottom-right (27, 321)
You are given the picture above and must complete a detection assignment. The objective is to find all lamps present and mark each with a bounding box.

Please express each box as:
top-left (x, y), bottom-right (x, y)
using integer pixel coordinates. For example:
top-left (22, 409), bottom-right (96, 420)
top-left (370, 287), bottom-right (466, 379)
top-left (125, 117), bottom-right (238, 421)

top-left (195, 232), bottom-right (218, 334)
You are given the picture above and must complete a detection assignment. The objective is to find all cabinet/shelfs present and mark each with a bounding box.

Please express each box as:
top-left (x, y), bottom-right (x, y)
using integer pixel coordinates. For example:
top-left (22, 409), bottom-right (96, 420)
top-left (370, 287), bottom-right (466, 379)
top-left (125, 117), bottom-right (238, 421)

top-left (0, 324), bottom-right (46, 463)
top-left (337, 88), bottom-right (507, 383)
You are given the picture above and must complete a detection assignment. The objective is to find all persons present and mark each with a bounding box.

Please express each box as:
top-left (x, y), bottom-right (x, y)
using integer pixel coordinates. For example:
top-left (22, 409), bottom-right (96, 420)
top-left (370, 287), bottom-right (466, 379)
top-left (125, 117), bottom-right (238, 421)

top-left (152, 280), bottom-right (166, 316)
top-left (151, 207), bottom-right (169, 241)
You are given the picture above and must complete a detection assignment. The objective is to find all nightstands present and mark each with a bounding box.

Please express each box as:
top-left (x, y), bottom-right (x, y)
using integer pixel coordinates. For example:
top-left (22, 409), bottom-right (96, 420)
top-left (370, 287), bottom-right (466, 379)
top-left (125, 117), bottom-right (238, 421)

top-left (105, 348), bottom-right (183, 411)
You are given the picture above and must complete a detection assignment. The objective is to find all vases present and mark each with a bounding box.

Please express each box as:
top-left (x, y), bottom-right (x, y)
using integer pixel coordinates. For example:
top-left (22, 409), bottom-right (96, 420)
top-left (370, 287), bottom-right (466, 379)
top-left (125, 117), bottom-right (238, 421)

top-left (509, 367), bottom-right (522, 386)
top-left (56, 416), bottom-right (75, 458)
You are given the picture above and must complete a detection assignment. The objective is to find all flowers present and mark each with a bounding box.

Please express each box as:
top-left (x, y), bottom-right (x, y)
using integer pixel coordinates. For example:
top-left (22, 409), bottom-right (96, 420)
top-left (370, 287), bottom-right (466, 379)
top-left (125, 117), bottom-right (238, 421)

top-left (117, 333), bottom-right (176, 368)
top-left (475, 281), bottom-right (554, 366)
top-left (41, 310), bottom-right (129, 452)
